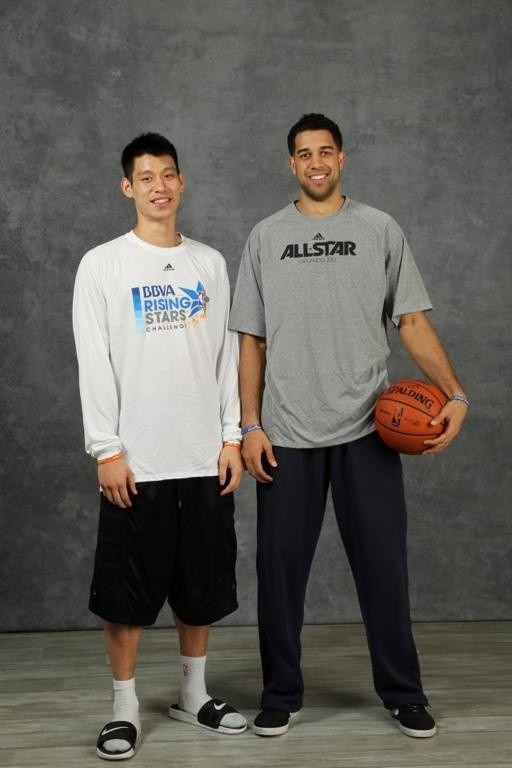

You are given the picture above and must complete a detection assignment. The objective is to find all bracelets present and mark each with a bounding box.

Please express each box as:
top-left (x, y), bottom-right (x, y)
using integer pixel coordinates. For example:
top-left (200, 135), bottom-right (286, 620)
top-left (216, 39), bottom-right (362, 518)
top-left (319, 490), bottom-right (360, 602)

top-left (96, 452), bottom-right (124, 465)
top-left (224, 442), bottom-right (242, 451)
top-left (450, 395), bottom-right (470, 408)
top-left (241, 423), bottom-right (262, 435)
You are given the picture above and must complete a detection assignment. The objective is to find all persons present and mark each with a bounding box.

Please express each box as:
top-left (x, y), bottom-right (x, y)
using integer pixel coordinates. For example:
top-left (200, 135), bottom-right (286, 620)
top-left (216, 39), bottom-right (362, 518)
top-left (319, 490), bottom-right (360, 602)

top-left (229, 113), bottom-right (471, 739)
top-left (72, 133), bottom-right (247, 761)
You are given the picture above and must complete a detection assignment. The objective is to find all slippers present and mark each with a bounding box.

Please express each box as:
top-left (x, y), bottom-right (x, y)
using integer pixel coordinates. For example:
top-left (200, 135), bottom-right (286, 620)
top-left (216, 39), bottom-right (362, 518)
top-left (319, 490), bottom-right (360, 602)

top-left (94, 718), bottom-right (145, 762)
top-left (167, 696), bottom-right (248, 735)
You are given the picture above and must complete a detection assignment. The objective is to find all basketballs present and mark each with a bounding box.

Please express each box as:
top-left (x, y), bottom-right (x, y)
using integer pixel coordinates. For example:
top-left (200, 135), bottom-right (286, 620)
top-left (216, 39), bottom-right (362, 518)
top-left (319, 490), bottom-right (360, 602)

top-left (377, 378), bottom-right (451, 455)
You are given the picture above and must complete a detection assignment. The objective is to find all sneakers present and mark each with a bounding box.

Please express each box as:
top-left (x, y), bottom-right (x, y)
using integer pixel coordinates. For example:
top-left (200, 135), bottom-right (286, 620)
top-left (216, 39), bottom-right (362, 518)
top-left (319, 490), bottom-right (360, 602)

top-left (389, 698), bottom-right (437, 739)
top-left (251, 701), bottom-right (298, 738)
